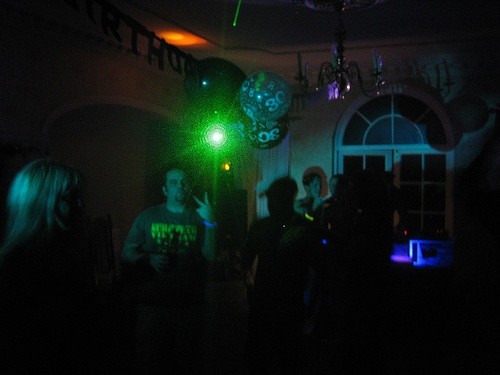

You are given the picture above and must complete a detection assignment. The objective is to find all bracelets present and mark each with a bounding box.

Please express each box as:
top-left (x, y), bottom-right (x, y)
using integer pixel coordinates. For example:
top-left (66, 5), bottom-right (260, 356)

top-left (204, 220), bottom-right (218, 229)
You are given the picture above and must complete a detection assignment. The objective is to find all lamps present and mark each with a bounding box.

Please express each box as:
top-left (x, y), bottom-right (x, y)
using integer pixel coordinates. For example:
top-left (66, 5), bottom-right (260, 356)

top-left (294, 0), bottom-right (384, 101)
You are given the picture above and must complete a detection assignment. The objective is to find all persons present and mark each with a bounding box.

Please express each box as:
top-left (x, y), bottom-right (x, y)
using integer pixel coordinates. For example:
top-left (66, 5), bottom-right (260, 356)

top-left (294, 162), bottom-right (413, 375)
top-left (239, 177), bottom-right (324, 375)
top-left (121, 160), bottom-right (218, 374)
top-left (0, 157), bottom-right (141, 375)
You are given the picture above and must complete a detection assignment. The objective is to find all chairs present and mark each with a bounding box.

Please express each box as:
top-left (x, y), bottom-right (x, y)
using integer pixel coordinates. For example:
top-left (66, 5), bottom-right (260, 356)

top-left (85, 213), bottom-right (115, 299)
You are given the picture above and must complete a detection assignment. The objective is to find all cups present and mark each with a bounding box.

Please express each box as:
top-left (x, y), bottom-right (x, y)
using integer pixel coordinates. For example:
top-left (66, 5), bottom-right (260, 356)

top-left (158, 241), bottom-right (171, 273)
